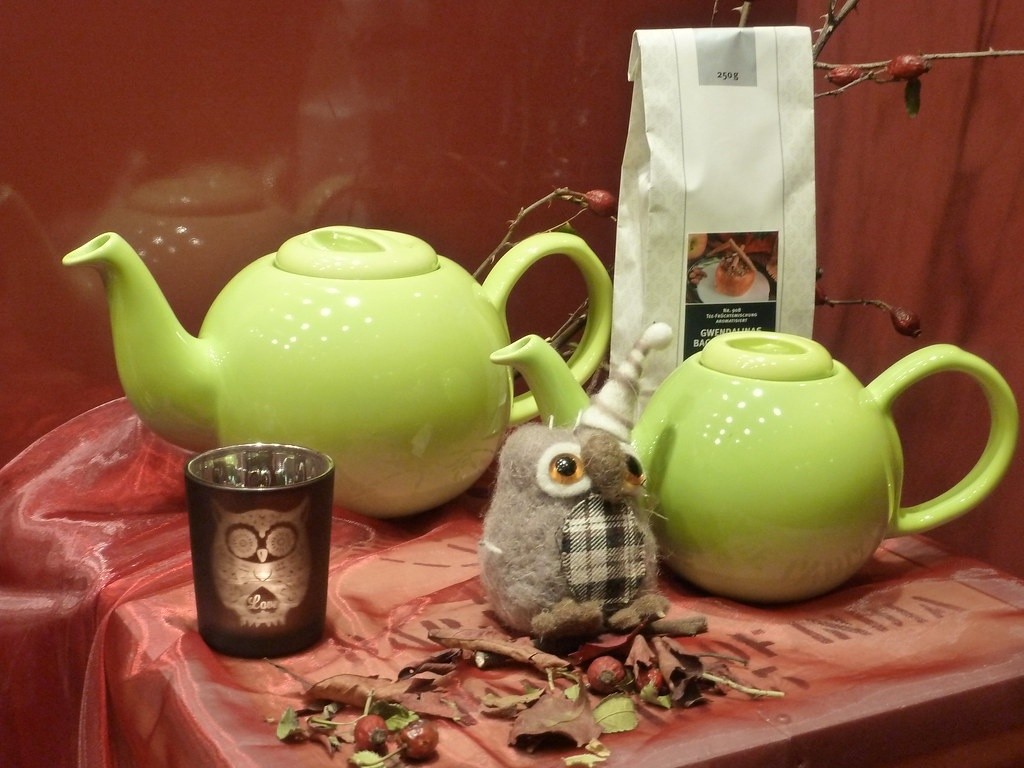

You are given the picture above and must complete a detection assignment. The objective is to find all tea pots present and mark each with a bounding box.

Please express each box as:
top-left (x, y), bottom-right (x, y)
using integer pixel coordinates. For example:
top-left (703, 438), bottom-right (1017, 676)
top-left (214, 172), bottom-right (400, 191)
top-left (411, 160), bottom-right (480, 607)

top-left (489, 331), bottom-right (1017, 598)
top-left (61, 226), bottom-right (613, 520)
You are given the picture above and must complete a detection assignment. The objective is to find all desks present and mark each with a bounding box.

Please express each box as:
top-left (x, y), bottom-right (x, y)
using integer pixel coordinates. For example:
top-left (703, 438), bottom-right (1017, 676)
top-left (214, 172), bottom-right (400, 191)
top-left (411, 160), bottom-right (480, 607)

top-left (2, 399), bottom-right (1024, 768)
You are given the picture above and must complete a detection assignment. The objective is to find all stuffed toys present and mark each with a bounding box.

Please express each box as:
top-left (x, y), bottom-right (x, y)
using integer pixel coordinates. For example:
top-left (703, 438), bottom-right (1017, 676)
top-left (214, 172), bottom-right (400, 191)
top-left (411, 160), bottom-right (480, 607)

top-left (478, 320), bottom-right (672, 640)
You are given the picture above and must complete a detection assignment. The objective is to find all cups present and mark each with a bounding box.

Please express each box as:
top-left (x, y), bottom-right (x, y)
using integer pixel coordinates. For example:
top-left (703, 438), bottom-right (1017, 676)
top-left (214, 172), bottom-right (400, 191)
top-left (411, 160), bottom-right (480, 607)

top-left (185, 442), bottom-right (334, 659)
